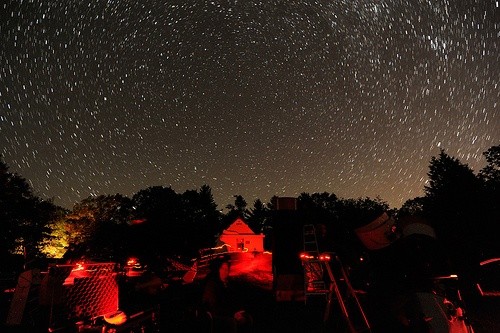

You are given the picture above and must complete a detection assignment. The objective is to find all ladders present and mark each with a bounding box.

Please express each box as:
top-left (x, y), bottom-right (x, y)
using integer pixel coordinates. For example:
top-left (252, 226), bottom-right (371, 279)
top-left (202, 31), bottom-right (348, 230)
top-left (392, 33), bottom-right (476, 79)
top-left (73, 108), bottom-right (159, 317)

top-left (301, 223), bottom-right (330, 306)
top-left (322, 255), bottom-right (377, 333)
